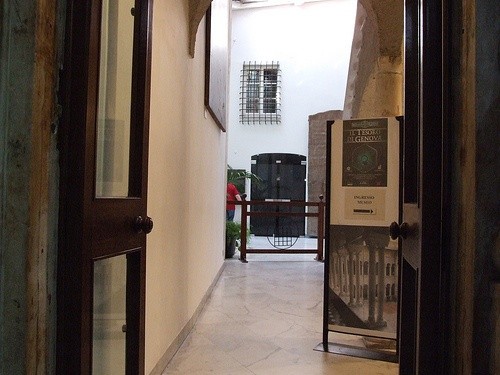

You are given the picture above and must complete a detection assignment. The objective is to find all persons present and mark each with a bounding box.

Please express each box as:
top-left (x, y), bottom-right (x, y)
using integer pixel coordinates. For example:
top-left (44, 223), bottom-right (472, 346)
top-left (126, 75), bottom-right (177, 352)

top-left (227, 182), bottom-right (241, 221)
